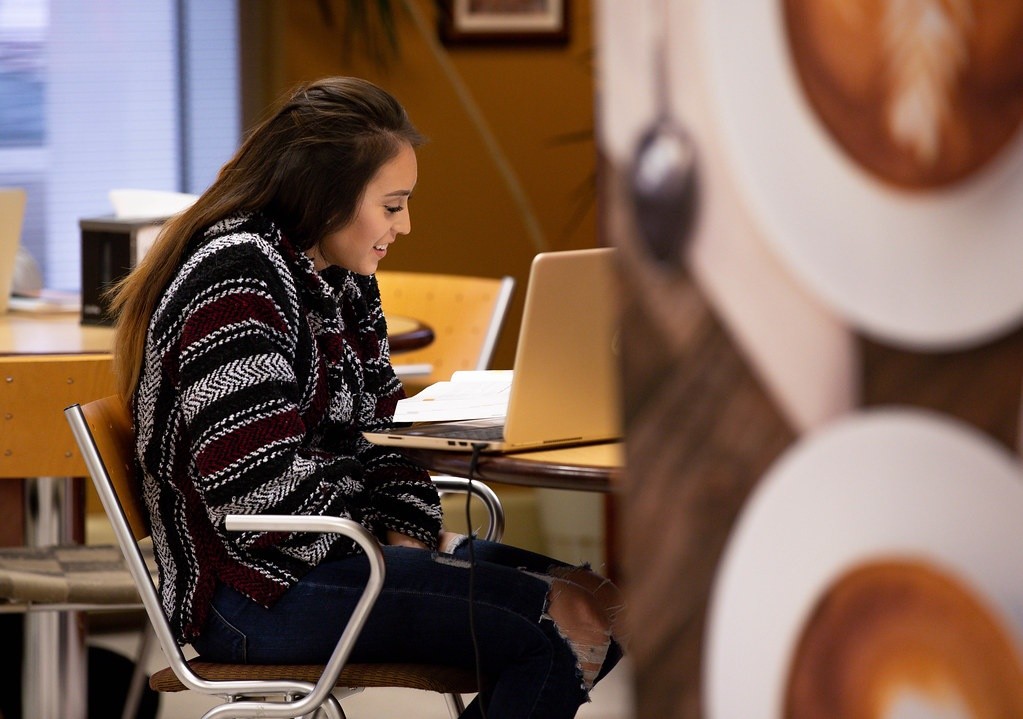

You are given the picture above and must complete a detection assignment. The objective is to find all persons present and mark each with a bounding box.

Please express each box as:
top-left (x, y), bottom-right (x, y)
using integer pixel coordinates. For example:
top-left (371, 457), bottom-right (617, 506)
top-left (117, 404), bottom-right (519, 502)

top-left (104, 77), bottom-right (626, 719)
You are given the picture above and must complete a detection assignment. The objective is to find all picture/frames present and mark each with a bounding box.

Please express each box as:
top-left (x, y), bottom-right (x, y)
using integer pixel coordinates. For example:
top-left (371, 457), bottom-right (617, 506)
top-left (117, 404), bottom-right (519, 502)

top-left (432, 0), bottom-right (571, 50)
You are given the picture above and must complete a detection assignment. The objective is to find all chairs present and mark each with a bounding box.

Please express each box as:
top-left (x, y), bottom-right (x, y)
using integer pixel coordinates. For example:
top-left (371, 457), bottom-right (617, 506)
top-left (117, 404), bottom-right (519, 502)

top-left (374, 269), bottom-right (517, 396)
top-left (0, 352), bottom-right (479, 719)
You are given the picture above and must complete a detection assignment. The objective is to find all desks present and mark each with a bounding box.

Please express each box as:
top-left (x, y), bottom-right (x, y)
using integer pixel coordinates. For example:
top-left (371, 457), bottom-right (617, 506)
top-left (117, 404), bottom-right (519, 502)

top-left (0, 311), bottom-right (121, 545)
top-left (421, 440), bottom-right (625, 689)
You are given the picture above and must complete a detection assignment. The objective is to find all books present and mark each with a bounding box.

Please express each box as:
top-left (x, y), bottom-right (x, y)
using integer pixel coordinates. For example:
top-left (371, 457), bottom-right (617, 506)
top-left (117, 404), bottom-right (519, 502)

top-left (393, 370), bottom-right (513, 421)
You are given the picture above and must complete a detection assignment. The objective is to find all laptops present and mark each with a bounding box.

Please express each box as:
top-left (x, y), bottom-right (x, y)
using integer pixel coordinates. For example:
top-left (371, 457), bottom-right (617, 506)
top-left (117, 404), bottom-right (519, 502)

top-left (361, 247), bottom-right (623, 453)
